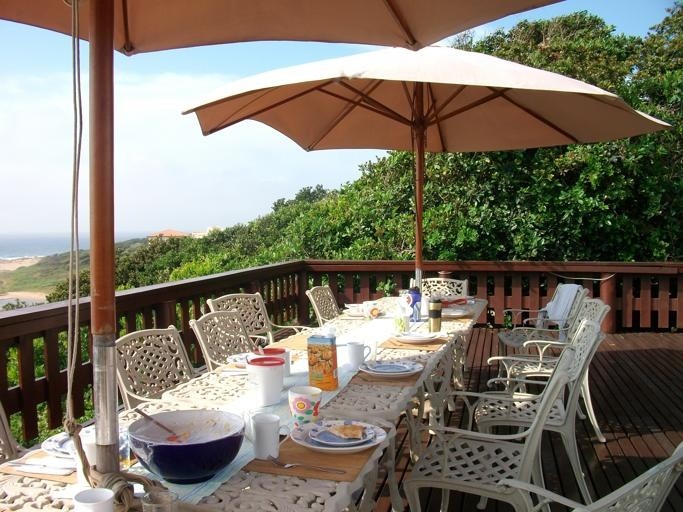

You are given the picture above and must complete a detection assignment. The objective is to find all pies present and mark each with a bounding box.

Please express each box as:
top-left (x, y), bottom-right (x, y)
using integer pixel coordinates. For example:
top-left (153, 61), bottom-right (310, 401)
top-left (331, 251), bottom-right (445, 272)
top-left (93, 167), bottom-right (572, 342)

top-left (326, 425), bottom-right (366, 441)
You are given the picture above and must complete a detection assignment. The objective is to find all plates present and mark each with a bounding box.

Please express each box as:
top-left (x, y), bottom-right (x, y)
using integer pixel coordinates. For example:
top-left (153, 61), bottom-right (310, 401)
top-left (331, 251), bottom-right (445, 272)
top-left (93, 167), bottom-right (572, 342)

top-left (227, 353), bottom-right (254, 365)
top-left (358, 360), bottom-right (425, 377)
top-left (41, 427), bottom-right (96, 459)
top-left (344, 303), bottom-right (363, 309)
top-left (290, 420), bottom-right (388, 454)
top-left (442, 307), bottom-right (470, 318)
top-left (392, 332), bottom-right (441, 344)
top-left (367, 362), bottom-right (414, 372)
top-left (400, 333), bottom-right (438, 338)
top-left (308, 423), bottom-right (375, 447)
top-left (343, 309), bottom-right (363, 317)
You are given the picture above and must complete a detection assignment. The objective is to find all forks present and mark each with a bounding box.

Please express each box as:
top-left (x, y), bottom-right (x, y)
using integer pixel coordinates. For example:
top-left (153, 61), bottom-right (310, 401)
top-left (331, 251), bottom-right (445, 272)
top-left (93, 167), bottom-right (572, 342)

top-left (266, 454), bottom-right (346, 475)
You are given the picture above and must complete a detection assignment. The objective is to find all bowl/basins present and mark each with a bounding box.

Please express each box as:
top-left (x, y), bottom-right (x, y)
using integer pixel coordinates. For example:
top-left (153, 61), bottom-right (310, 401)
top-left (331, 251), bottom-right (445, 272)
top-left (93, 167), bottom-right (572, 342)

top-left (251, 413), bottom-right (291, 461)
top-left (126, 410), bottom-right (245, 484)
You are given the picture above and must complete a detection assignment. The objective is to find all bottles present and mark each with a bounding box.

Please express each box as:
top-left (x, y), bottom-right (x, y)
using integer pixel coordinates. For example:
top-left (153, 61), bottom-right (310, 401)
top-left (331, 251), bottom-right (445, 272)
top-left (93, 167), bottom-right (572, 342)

top-left (407, 287), bottom-right (423, 322)
top-left (428, 298), bottom-right (442, 334)
top-left (306, 335), bottom-right (339, 391)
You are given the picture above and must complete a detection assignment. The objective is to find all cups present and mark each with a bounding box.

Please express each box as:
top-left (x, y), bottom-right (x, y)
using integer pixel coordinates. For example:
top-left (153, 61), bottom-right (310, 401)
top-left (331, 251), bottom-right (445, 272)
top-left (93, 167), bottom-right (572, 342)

top-left (119, 418), bottom-right (130, 472)
top-left (347, 342), bottom-right (371, 371)
top-left (140, 491), bottom-right (178, 511)
top-left (74, 488), bottom-right (114, 512)
top-left (396, 316), bottom-right (415, 336)
top-left (363, 301), bottom-right (379, 319)
top-left (67, 434), bottom-right (97, 486)
top-left (288, 387), bottom-right (322, 421)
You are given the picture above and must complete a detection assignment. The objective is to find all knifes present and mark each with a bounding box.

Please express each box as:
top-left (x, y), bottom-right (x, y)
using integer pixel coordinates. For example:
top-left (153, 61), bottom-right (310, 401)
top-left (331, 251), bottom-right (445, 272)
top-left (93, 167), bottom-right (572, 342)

top-left (57, 435), bottom-right (71, 447)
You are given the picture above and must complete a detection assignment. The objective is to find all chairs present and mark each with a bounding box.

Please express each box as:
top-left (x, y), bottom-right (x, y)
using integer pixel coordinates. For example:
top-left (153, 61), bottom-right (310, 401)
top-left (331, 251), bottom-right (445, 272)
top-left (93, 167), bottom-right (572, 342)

top-left (470, 319), bottom-right (607, 509)
top-left (206, 292), bottom-right (311, 347)
top-left (114, 325), bottom-right (204, 410)
top-left (495, 439), bottom-right (683, 512)
top-left (506, 295), bottom-right (612, 442)
top-left (189, 309), bottom-right (260, 370)
top-left (304, 284), bottom-right (341, 326)
top-left (401, 345), bottom-right (580, 512)
top-left (490, 282), bottom-right (590, 383)
top-left (409, 275), bottom-right (471, 297)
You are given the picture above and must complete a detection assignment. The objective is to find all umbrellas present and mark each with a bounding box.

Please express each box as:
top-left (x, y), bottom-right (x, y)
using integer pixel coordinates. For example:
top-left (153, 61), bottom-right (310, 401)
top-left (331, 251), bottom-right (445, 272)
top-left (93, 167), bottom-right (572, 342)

top-left (181, 42), bottom-right (677, 289)
top-left (3, 2), bottom-right (566, 475)
